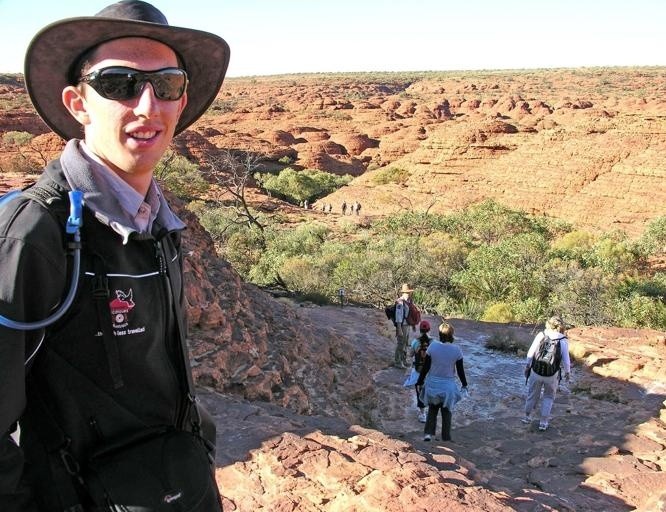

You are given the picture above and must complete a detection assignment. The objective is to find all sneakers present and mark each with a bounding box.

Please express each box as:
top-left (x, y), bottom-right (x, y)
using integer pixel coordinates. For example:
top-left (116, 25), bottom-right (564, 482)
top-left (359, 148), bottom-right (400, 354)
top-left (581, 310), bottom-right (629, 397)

top-left (539, 421), bottom-right (550, 431)
top-left (521, 416), bottom-right (531, 423)
top-left (403, 359), bottom-right (412, 367)
top-left (396, 361), bottom-right (405, 369)
top-left (423, 432), bottom-right (433, 442)
top-left (418, 412), bottom-right (426, 422)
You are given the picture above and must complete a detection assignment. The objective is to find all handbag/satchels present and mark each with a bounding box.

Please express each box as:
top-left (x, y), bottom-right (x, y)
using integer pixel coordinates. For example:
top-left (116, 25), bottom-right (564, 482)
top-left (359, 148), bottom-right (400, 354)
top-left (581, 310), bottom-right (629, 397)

top-left (51, 404), bottom-right (228, 512)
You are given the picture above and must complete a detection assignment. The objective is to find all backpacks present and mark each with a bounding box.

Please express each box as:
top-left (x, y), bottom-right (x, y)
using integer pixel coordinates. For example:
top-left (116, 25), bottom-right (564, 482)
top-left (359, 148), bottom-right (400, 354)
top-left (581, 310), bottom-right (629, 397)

top-left (405, 301), bottom-right (421, 325)
top-left (385, 303), bottom-right (397, 326)
top-left (413, 337), bottom-right (432, 372)
top-left (532, 331), bottom-right (566, 378)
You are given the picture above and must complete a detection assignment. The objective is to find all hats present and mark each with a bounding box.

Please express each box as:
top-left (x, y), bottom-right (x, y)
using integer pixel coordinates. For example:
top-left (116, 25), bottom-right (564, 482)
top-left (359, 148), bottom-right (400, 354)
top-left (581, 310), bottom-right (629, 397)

top-left (420, 320), bottom-right (431, 332)
top-left (398, 282), bottom-right (415, 293)
top-left (19, 0), bottom-right (232, 144)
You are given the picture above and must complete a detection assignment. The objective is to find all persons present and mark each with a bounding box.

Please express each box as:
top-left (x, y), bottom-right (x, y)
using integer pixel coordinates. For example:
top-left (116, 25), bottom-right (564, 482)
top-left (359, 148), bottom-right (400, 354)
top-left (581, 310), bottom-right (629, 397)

top-left (416, 323), bottom-right (469, 442)
top-left (1, 2), bottom-right (231, 512)
top-left (394, 284), bottom-right (415, 370)
top-left (402, 321), bottom-right (436, 422)
top-left (520, 315), bottom-right (571, 431)
top-left (304, 198), bottom-right (360, 215)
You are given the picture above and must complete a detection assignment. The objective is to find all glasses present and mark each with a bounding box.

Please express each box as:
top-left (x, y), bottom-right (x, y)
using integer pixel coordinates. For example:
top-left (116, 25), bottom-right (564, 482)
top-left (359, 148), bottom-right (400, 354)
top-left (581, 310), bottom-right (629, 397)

top-left (75, 65), bottom-right (190, 104)
